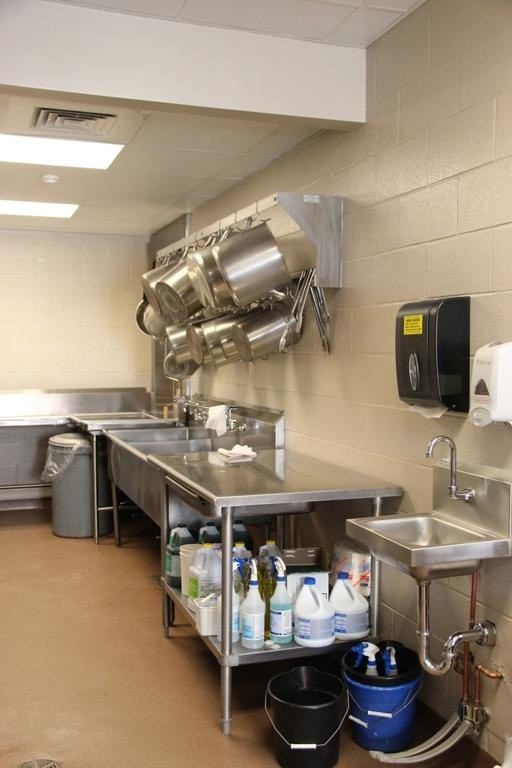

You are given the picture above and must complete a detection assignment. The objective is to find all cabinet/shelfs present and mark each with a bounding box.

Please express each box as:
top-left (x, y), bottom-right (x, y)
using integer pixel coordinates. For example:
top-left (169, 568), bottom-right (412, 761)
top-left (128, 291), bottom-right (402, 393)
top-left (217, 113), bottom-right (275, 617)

top-left (147, 448), bottom-right (406, 736)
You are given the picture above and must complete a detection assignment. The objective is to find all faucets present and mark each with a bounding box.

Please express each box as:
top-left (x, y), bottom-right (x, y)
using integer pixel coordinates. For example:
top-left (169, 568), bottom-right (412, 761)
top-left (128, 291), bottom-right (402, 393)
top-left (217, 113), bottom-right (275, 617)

top-left (225, 406), bottom-right (238, 430)
top-left (192, 405), bottom-right (205, 421)
top-left (425, 432), bottom-right (474, 505)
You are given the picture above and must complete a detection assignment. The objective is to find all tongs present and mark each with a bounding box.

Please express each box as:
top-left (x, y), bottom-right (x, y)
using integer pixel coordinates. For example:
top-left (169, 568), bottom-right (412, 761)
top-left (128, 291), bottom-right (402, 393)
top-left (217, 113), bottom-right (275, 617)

top-left (278, 267), bottom-right (332, 355)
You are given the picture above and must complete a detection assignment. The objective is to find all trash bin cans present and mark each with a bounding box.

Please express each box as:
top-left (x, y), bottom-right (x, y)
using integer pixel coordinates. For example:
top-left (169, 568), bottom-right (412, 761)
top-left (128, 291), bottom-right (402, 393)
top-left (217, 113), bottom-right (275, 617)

top-left (48, 433), bottom-right (113, 539)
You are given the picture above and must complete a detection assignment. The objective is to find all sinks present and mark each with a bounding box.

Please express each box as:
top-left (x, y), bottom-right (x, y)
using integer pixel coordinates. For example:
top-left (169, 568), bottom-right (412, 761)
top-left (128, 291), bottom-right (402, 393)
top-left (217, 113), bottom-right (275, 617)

top-left (74, 411), bottom-right (158, 426)
top-left (104, 422), bottom-right (213, 443)
top-left (344, 508), bottom-right (512, 579)
top-left (125, 436), bottom-right (250, 459)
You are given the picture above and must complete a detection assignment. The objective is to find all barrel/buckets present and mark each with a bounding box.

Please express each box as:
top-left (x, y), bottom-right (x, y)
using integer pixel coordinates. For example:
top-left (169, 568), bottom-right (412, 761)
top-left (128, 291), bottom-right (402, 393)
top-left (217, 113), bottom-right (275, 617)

top-left (193, 594), bottom-right (222, 637)
top-left (256, 540), bottom-right (282, 641)
top-left (196, 520), bottom-right (222, 542)
top-left (186, 542), bottom-right (222, 614)
top-left (339, 638), bottom-right (423, 753)
top-left (230, 519), bottom-right (253, 551)
top-left (163, 523), bottom-right (196, 588)
top-left (263, 664), bottom-right (351, 768)
top-left (330, 571), bottom-right (372, 642)
top-left (294, 576), bottom-right (337, 647)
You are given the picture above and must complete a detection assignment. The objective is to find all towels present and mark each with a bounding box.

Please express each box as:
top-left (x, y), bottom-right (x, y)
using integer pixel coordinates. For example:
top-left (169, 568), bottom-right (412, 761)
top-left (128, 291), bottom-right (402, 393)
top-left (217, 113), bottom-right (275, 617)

top-left (204, 405), bottom-right (230, 437)
top-left (218, 443), bottom-right (257, 463)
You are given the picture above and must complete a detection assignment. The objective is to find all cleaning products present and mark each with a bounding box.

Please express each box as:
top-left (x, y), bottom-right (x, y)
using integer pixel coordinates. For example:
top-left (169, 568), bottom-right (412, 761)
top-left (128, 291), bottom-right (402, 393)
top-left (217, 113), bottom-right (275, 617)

top-left (267, 555), bottom-right (293, 644)
top-left (347, 641), bottom-right (401, 677)
top-left (217, 556), bottom-right (247, 643)
top-left (239, 556), bottom-right (266, 649)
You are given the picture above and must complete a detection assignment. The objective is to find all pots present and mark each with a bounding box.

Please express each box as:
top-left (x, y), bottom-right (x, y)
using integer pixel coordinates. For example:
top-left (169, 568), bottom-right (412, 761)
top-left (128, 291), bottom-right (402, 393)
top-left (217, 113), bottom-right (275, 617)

top-left (136, 225), bottom-right (302, 381)
